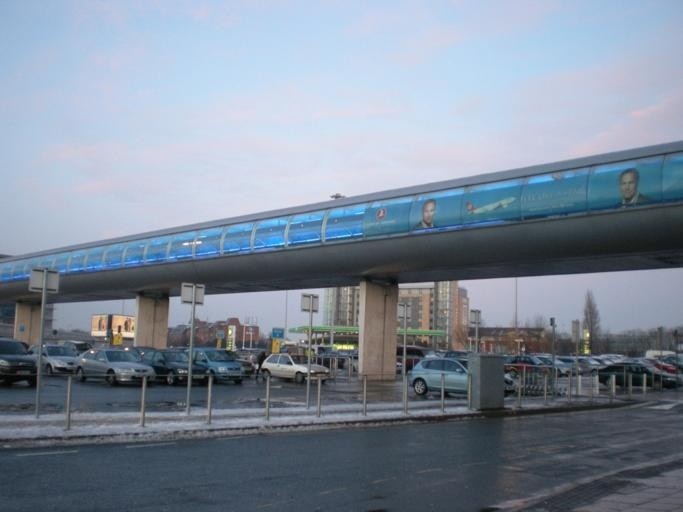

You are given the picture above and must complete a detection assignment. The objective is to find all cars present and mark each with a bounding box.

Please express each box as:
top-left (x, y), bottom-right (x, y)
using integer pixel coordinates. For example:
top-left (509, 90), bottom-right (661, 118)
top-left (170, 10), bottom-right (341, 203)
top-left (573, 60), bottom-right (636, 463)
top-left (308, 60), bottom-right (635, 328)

top-left (396, 345), bottom-right (683, 399)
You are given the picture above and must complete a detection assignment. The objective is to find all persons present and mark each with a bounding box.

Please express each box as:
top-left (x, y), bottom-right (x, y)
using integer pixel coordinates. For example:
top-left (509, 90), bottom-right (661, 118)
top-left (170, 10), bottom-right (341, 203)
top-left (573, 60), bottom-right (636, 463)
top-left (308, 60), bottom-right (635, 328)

top-left (252, 351), bottom-right (267, 380)
top-left (412, 199), bottom-right (437, 229)
top-left (615, 168), bottom-right (651, 206)
